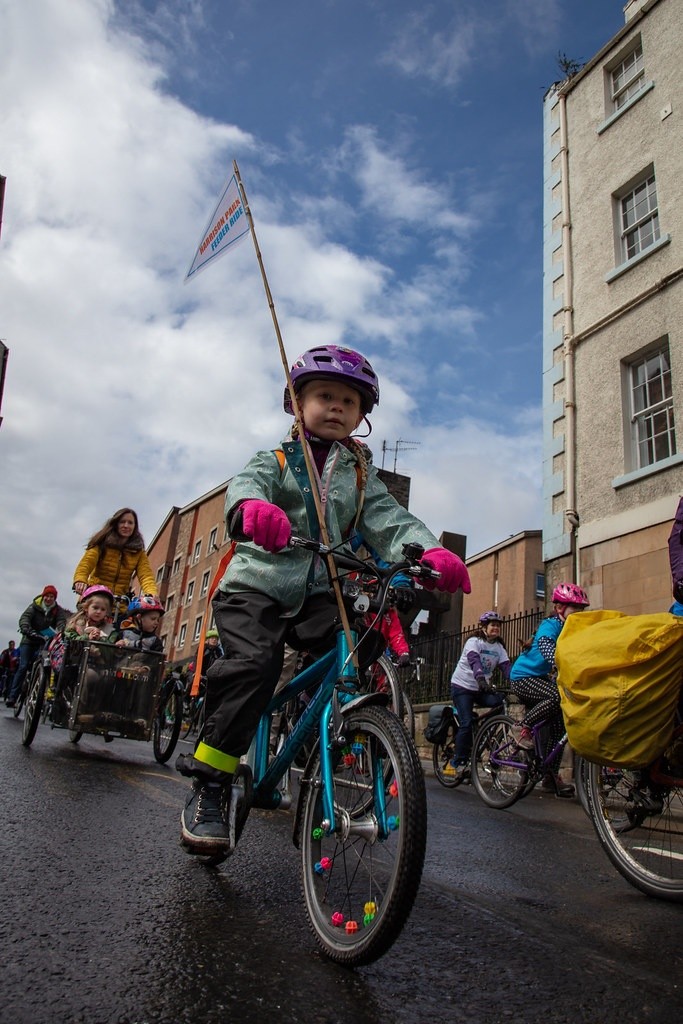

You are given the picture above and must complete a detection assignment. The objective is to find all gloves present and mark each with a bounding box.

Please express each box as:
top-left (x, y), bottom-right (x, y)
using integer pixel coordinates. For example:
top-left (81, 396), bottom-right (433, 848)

top-left (477, 678), bottom-right (491, 693)
top-left (237, 498), bottom-right (291, 554)
top-left (398, 653), bottom-right (410, 668)
top-left (386, 585), bottom-right (416, 614)
top-left (414, 548), bottom-right (471, 595)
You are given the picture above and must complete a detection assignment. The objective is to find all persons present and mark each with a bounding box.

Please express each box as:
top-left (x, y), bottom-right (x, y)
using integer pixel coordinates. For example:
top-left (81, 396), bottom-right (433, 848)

top-left (0, 584), bottom-right (67, 706)
top-left (507, 582), bottom-right (590, 793)
top-left (443, 611), bottom-right (511, 779)
top-left (50, 508), bottom-right (165, 735)
top-left (180, 343), bottom-right (468, 850)
top-left (668, 497), bottom-right (683, 604)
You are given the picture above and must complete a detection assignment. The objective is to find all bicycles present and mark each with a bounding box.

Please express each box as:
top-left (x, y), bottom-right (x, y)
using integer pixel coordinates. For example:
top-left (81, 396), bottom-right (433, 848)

top-left (433, 679), bottom-right (683, 900)
top-left (181, 509), bottom-right (443, 966)
top-left (0, 585), bottom-right (425, 819)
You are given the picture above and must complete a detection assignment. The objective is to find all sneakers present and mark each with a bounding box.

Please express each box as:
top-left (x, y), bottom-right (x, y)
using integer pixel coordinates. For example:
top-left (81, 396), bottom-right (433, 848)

top-left (508, 722), bottom-right (533, 749)
top-left (456, 766), bottom-right (470, 775)
top-left (281, 704), bottom-right (315, 766)
top-left (181, 777), bottom-right (231, 847)
top-left (541, 775), bottom-right (575, 794)
top-left (166, 716), bottom-right (172, 724)
top-left (482, 760), bottom-right (492, 773)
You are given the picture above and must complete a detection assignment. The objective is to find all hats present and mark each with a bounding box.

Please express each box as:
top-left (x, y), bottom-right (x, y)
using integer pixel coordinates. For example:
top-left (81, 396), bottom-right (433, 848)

top-left (206, 630), bottom-right (219, 639)
top-left (42, 586), bottom-right (57, 600)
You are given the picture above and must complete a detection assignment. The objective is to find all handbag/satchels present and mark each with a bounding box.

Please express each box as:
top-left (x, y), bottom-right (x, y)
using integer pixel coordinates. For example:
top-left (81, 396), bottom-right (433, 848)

top-left (422, 705), bottom-right (453, 744)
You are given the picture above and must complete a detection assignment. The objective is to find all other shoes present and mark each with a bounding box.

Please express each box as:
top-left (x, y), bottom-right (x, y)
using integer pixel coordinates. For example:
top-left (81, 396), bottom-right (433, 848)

top-left (338, 759), bottom-right (345, 768)
top-left (44, 689), bottom-right (55, 701)
top-left (5, 700), bottom-right (14, 708)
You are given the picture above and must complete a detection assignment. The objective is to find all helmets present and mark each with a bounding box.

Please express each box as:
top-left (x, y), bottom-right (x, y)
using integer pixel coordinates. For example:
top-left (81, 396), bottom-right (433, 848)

top-left (284, 345), bottom-right (379, 416)
top-left (80, 585), bottom-right (114, 604)
top-left (551, 582), bottom-right (590, 605)
top-left (127, 594), bottom-right (165, 616)
top-left (479, 611), bottom-right (501, 621)
